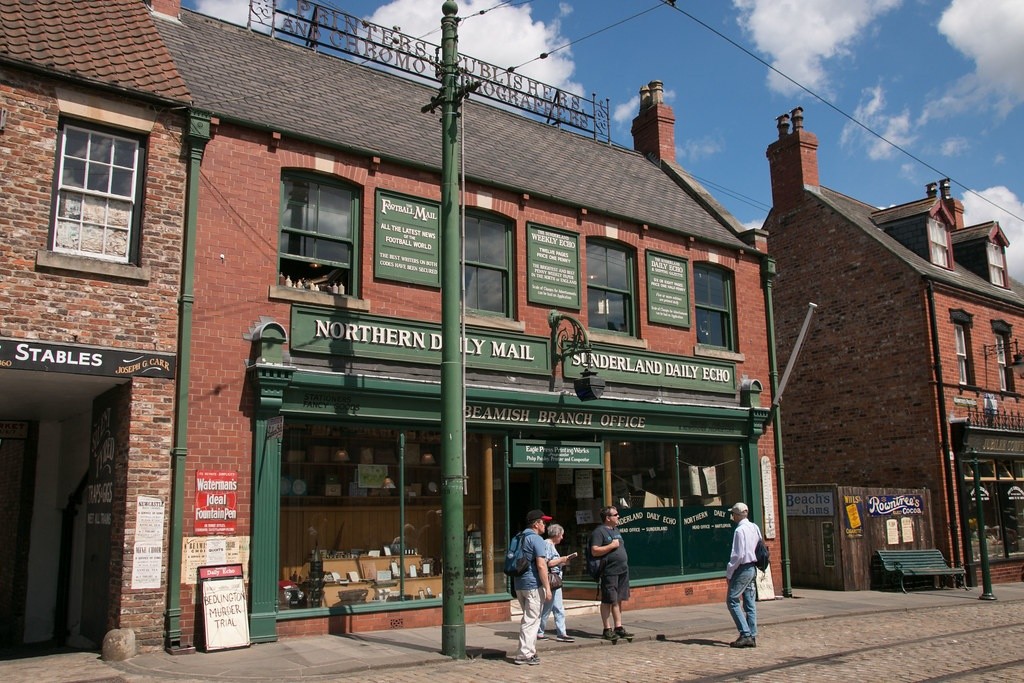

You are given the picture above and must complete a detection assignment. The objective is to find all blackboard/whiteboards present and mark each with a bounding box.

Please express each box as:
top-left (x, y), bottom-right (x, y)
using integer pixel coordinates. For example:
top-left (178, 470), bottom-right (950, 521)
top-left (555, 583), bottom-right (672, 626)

top-left (202, 579), bottom-right (250, 650)
top-left (755, 562), bottom-right (776, 601)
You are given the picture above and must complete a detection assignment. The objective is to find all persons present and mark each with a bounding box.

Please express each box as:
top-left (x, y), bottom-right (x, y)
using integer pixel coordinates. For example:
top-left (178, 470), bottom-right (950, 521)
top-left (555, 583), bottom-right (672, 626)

top-left (726, 502), bottom-right (762, 648)
top-left (590, 506), bottom-right (635, 641)
top-left (502, 509), bottom-right (552, 665)
top-left (536, 524), bottom-right (578, 643)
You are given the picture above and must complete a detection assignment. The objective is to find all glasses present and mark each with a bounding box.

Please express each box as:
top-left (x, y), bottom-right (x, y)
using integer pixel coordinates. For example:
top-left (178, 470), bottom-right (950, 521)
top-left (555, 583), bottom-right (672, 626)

top-left (609, 513), bottom-right (619, 517)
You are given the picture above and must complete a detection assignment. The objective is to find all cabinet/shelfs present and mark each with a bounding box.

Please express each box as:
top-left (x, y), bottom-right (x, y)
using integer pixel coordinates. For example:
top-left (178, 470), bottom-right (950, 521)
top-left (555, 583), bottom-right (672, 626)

top-left (280, 434), bottom-right (452, 506)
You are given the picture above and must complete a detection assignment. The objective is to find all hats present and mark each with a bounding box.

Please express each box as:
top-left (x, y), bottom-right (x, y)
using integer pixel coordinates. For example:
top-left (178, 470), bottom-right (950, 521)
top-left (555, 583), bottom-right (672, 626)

top-left (727, 502), bottom-right (749, 516)
top-left (527, 509), bottom-right (553, 522)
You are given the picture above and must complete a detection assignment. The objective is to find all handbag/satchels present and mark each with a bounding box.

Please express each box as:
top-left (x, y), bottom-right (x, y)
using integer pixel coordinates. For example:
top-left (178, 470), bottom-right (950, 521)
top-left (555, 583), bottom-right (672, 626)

top-left (547, 567), bottom-right (563, 589)
top-left (755, 524), bottom-right (770, 572)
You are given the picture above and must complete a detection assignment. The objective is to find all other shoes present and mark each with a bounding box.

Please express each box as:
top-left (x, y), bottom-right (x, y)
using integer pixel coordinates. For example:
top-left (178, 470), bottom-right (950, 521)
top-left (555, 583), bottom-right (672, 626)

top-left (514, 655), bottom-right (541, 665)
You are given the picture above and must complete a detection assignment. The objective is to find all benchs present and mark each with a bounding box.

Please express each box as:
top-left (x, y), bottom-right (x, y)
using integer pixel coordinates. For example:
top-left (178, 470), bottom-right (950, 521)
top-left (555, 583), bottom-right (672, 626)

top-left (875, 548), bottom-right (969, 594)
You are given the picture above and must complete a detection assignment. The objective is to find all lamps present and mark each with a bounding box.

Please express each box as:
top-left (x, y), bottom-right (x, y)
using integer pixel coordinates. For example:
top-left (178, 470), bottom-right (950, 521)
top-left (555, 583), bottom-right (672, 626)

top-left (984, 340), bottom-right (1024, 378)
top-left (546, 309), bottom-right (604, 403)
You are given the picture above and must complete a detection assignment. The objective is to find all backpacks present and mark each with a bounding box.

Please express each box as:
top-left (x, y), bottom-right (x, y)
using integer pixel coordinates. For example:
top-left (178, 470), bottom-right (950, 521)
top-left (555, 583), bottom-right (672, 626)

top-left (585, 528), bottom-right (613, 580)
top-left (504, 532), bottom-right (538, 576)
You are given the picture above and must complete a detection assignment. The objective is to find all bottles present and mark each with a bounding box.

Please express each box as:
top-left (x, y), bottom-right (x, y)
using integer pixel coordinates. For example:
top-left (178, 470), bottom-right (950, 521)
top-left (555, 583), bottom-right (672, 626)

top-left (292, 279), bottom-right (319, 291)
top-left (332, 283), bottom-right (339, 294)
top-left (279, 273), bottom-right (285, 286)
top-left (338, 283), bottom-right (345, 295)
top-left (285, 276), bottom-right (292, 288)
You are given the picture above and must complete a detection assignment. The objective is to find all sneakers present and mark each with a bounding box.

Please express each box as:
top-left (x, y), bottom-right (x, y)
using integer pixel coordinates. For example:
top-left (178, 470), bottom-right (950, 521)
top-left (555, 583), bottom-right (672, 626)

top-left (615, 628), bottom-right (635, 639)
top-left (729, 636), bottom-right (757, 647)
top-left (602, 628), bottom-right (620, 640)
top-left (557, 634), bottom-right (576, 642)
top-left (537, 635), bottom-right (549, 641)
